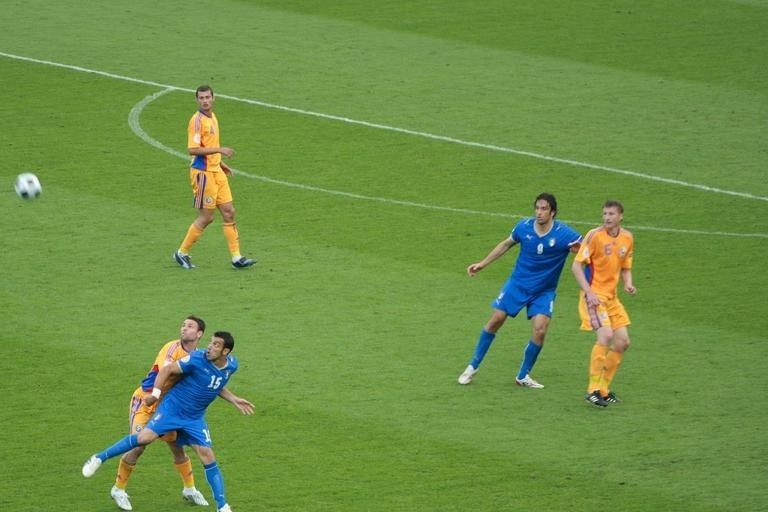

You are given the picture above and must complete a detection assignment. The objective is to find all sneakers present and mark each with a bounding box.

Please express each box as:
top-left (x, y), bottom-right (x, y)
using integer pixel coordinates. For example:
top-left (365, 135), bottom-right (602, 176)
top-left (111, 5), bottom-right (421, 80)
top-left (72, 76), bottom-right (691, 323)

top-left (82, 454), bottom-right (102, 477)
top-left (231, 257), bottom-right (256, 269)
top-left (181, 486), bottom-right (210, 508)
top-left (515, 373), bottom-right (545, 390)
top-left (172, 250), bottom-right (196, 270)
top-left (111, 485), bottom-right (132, 511)
top-left (583, 389), bottom-right (609, 408)
top-left (602, 391), bottom-right (622, 406)
top-left (216, 503), bottom-right (234, 512)
top-left (458, 365), bottom-right (479, 385)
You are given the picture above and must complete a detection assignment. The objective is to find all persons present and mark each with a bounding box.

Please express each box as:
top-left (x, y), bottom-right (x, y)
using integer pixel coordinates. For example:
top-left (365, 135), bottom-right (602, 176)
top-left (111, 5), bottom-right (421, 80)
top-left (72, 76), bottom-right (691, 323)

top-left (110, 316), bottom-right (255, 510)
top-left (456, 192), bottom-right (586, 389)
top-left (572, 201), bottom-right (638, 409)
top-left (82, 332), bottom-right (238, 512)
top-left (172, 86), bottom-right (258, 269)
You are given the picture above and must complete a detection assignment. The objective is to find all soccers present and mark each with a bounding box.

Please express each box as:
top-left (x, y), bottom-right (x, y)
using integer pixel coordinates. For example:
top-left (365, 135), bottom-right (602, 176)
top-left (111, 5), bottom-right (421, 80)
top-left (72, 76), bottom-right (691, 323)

top-left (14, 172), bottom-right (42, 199)
